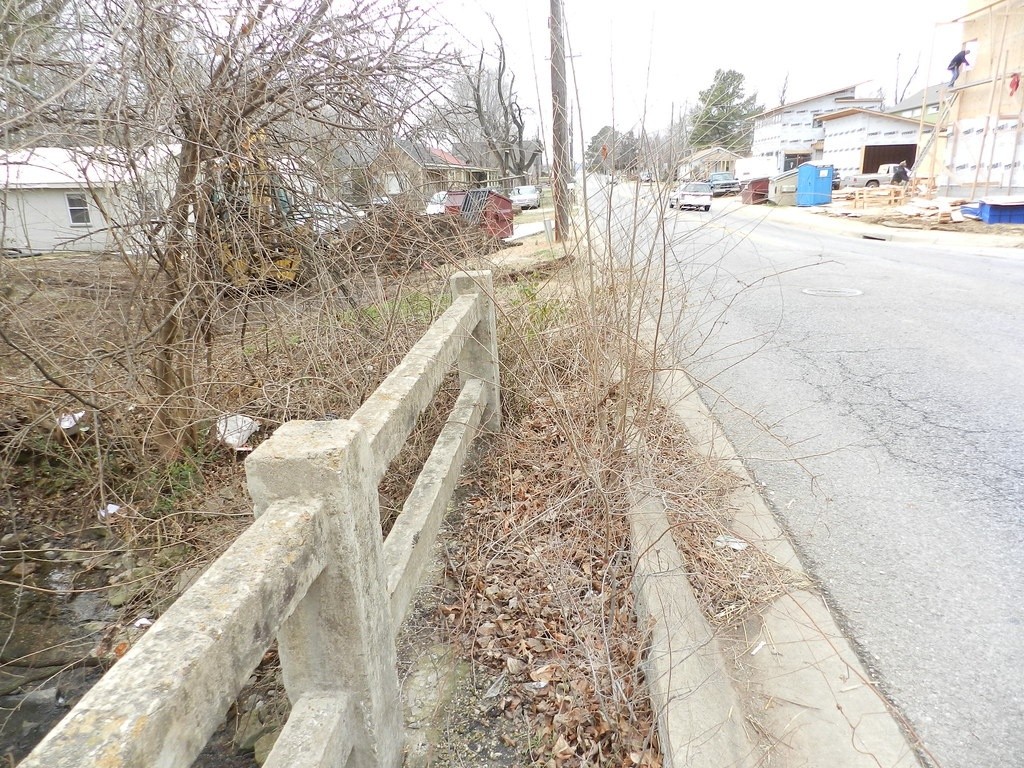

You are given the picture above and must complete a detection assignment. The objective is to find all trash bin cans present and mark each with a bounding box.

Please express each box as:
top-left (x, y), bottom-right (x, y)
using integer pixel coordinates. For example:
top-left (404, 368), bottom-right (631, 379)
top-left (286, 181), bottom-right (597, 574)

top-left (796, 159), bottom-right (834, 207)
top-left (445, 188), bottom-right (515, 239)
top-left (740, 176), bottom-right (771, 205)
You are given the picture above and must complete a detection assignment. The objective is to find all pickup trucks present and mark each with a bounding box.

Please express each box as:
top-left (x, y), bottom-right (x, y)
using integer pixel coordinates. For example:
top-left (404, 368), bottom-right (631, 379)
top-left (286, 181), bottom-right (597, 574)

top-left (844, 163), bottom-right (912, 189)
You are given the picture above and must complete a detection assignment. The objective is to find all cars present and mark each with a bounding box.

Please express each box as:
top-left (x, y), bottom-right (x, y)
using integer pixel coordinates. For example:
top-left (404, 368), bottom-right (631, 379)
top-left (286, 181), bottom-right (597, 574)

top-left (832, 168), bottom-right (840, 189)
top-left (508, 186), bottom-right (540, 209)
top-left (371, 197), bottom-right (389, 206)
top-left (604, 174), bottom-right (618, 184)
top-left (297, 203), bottom-right (364, 233)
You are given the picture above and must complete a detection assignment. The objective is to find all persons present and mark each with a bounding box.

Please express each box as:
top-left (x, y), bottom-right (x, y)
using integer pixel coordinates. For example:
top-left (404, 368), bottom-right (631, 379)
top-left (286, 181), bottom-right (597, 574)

top-left (890, 159), bottom-right (910, 204)
top-left (947, 49), bottom-right (971, 88)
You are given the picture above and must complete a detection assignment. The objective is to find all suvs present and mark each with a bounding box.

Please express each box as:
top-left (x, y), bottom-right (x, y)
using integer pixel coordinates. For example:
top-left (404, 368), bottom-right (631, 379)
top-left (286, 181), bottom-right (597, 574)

top-left (669, 182), bottom-right (712, 211)
top-left (425, 191), bottom-right (448, 216)
top-left (708, 172), bottom-right (740, 196)
